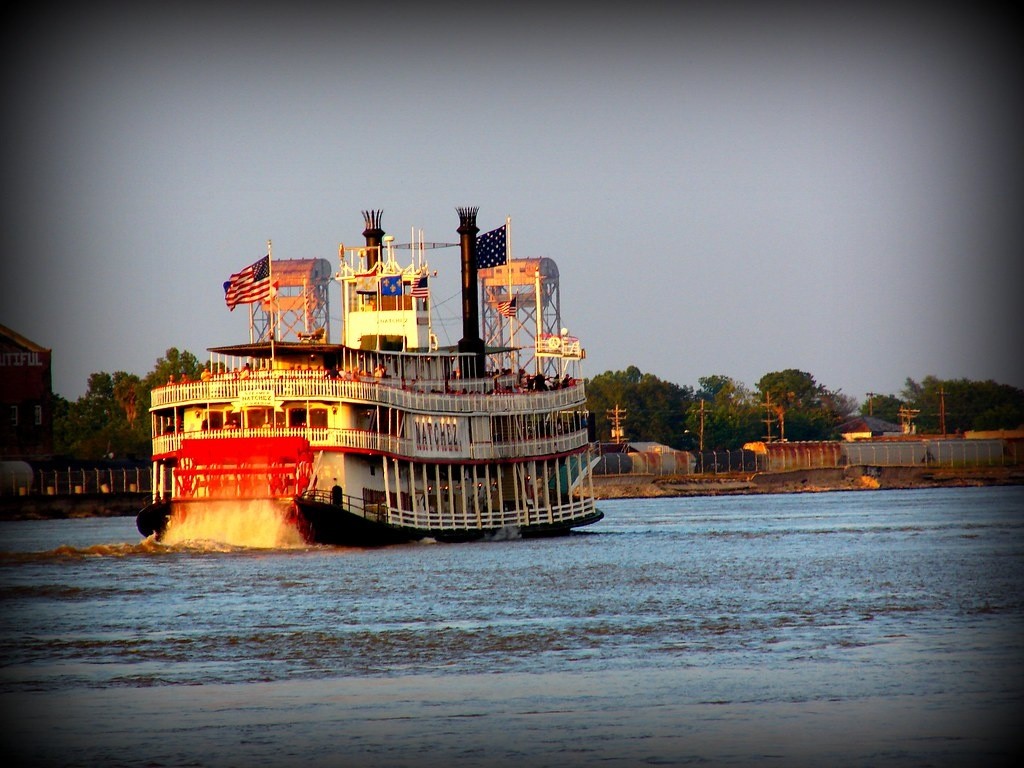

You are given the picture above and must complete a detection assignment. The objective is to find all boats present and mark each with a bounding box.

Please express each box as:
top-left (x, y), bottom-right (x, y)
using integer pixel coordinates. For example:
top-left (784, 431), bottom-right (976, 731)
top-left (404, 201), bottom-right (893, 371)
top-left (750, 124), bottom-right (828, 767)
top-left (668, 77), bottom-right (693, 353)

top-left (133, 203), bottom-right (608, 553)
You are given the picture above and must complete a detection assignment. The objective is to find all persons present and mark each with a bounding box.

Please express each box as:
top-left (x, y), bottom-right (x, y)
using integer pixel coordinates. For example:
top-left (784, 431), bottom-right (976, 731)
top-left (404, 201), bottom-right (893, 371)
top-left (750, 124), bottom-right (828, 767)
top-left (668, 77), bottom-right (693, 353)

top-left (289, 363), bottom-right (391, 381)
top-left (166, 375), bottom-right (176, 385)
top-left (536, 411), bottom-right (587, 438)
top-left (219, 363), bottom-right (251, 380)
top-left (200, 368), bottom-right (212, 383)
top-left (180, 372), bottom-right (191, 384)
top-left (401, 367), bottom-right (578, 396)
top-left (166, 420), bottom-right (174, 432)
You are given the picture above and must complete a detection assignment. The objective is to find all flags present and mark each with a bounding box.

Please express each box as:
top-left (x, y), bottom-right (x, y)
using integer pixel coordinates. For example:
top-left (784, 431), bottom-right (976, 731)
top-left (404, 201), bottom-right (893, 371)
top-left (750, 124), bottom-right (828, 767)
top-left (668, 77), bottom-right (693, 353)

top-left (223, 254), bottom-right (278, 312)
top-left (475, 224), bottom-right (507, 270)
top-left (409, 277), bottom-right (428, 298)
top-left (381, 276), bottom-right (402, 297)
top-left (498, 297), bottom-right (515, 318)
top-left (355, 264), bottom-right (378, 296)
